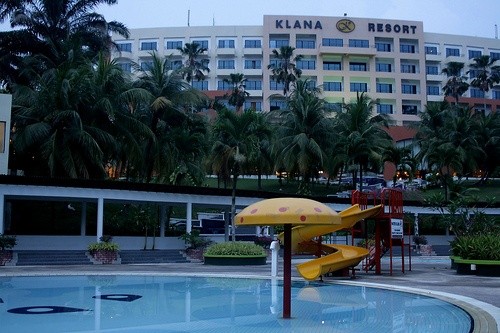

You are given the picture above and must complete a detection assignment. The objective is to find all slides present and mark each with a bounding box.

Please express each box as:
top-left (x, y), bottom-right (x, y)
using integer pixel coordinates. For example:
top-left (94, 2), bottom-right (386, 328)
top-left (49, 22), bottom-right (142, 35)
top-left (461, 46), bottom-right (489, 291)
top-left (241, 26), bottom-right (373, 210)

top-left (276, 204), bottom-right (382, 280)
